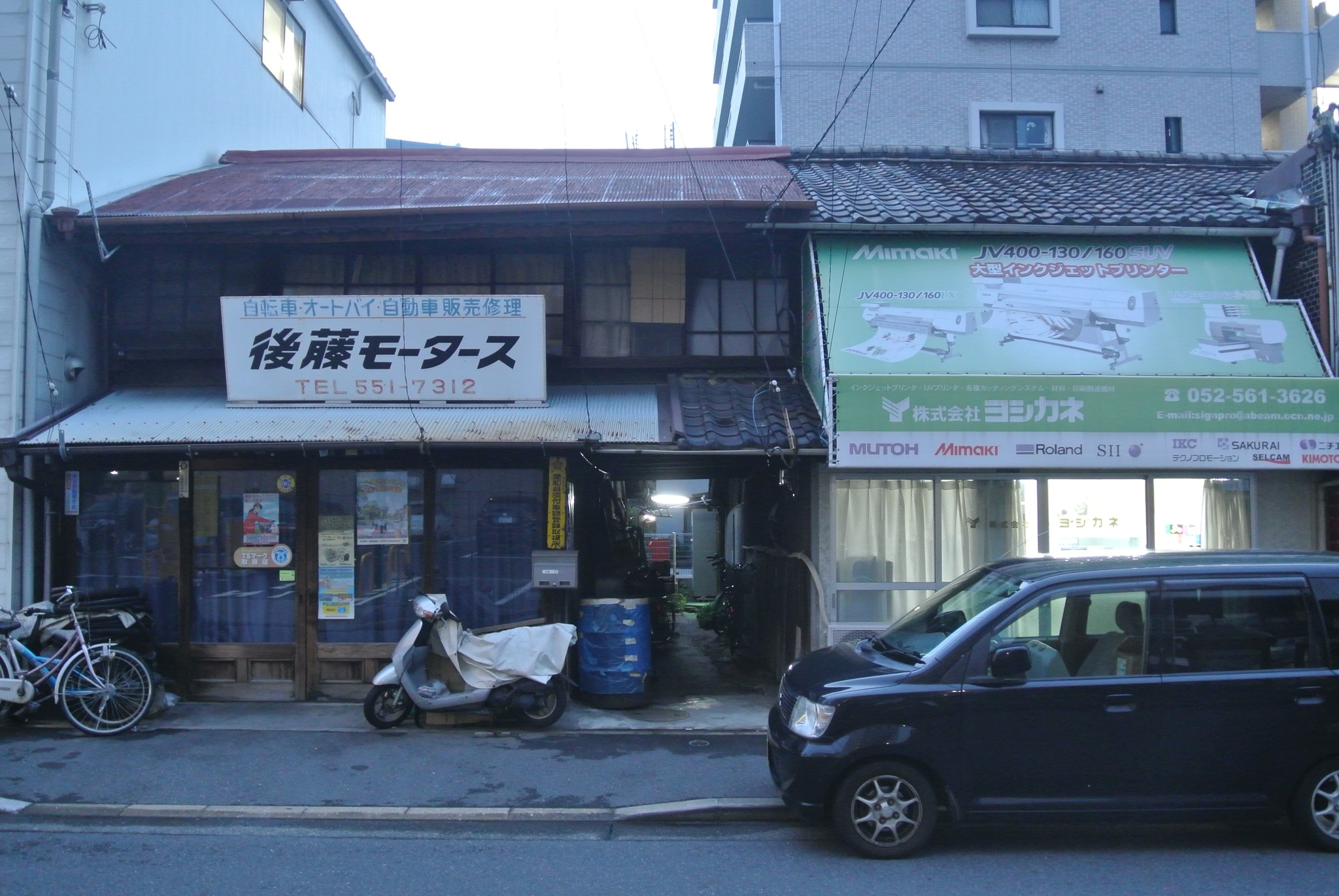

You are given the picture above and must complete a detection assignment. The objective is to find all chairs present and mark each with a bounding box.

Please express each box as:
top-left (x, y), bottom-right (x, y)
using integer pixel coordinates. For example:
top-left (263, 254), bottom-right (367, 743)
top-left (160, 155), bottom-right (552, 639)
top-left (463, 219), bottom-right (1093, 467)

top-left (1075, 599), bottom-right (1144, 677)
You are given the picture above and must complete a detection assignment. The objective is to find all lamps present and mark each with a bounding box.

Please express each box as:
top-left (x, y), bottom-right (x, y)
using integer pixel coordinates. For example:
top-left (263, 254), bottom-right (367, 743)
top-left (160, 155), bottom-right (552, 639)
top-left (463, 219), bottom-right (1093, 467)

top-left (1095, 84), bottom-right (1104, 94)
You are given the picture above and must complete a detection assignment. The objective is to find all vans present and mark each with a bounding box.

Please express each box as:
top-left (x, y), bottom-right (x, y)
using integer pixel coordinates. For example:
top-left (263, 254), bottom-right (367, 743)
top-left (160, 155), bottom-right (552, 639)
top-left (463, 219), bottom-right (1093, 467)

top-left (765, 549), bottom-right (1339, 853)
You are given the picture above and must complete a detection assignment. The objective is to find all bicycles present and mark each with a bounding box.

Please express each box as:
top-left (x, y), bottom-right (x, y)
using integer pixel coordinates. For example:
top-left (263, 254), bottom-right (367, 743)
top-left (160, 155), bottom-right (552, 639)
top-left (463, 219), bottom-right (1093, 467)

top-left (704, 553), bottom-right (759, 649)
top-left (0, 586), bottom-right (155, 736)
top-left (645, 571), bottom-right (679, 642)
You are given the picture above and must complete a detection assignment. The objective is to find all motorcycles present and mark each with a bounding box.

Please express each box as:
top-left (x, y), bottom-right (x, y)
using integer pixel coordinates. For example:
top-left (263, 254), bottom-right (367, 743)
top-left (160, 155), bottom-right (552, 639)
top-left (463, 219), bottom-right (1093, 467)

top-left (364, 562), bottom-right (584, 729)
